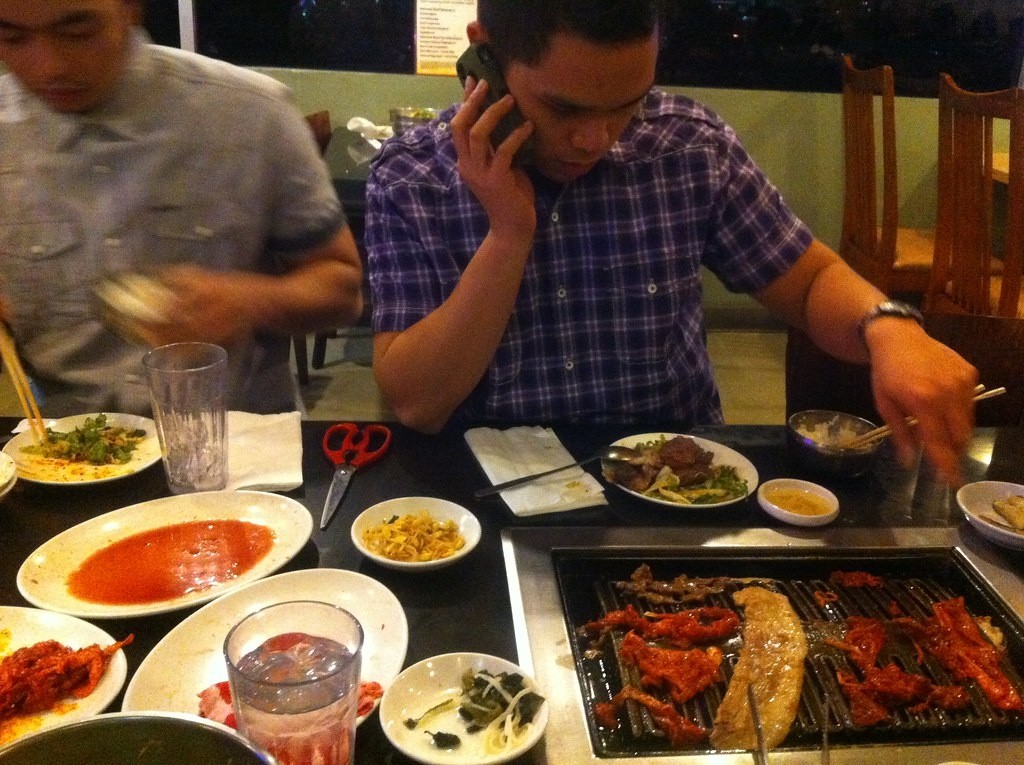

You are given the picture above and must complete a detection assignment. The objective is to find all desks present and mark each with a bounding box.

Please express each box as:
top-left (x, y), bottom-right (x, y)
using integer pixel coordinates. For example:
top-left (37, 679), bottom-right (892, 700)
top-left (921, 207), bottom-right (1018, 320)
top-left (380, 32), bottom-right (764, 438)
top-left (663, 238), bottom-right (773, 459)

top-left (312, 126), bottom-right (383, 369)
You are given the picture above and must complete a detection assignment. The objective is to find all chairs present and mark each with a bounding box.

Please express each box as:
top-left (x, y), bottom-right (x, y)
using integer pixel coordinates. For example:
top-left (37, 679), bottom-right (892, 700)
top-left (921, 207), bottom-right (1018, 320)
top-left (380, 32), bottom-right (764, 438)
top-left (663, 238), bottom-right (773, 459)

top-left (839, 54), bottom-right (1004, 297)
top-left (923, 74), bottom-right (1024, 318)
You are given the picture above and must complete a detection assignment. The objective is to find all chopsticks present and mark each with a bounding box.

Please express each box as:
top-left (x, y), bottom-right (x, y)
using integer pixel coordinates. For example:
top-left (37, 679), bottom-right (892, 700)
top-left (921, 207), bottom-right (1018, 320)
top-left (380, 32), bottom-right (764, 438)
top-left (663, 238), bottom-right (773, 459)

top-left (844, 384), bottom-right (1006, 445)
top-left (0, 322), bottom-right (51, 446)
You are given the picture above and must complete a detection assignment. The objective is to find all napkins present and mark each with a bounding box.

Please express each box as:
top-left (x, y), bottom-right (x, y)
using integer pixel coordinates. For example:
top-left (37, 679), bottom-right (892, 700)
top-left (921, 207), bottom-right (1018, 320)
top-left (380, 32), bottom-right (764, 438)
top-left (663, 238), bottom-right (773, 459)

top-left (465, 425), bottom-right (609, 518)
top-left (346, 116), bottom-right (392, 139)
top-left (223, 410), bottom-right (302, 492)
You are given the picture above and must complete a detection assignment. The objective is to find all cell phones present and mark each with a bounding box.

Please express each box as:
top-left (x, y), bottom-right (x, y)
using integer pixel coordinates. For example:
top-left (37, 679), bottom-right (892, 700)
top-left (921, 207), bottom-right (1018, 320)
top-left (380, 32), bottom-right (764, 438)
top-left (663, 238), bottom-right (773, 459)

top-left (455, 41), bottom-right (535, 165)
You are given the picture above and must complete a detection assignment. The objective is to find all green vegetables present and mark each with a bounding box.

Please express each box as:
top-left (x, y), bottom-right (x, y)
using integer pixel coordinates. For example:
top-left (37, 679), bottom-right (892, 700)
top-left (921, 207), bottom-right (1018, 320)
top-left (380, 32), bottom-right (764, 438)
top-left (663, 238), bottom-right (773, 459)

top-left (38, 412), bottom-right (147, 464)
top-left (401, 672), bottom-right (546, 750)
top-left (635, 434), bottom-right (749, 503)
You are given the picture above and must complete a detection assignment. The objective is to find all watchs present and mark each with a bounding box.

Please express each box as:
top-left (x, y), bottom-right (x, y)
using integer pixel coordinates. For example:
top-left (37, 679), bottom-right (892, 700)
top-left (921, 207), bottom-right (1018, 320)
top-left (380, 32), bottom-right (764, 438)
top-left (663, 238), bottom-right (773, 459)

top-left (858, 300), bottom-right (926, 344)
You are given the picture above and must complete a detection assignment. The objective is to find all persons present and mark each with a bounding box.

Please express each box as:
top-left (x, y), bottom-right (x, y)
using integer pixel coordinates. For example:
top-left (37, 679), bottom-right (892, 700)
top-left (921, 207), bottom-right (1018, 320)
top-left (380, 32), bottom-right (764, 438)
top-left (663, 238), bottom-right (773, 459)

top-left (0, 0), bottom-right (365, 420)
top-left (365, 0), bottom-right (979, 489)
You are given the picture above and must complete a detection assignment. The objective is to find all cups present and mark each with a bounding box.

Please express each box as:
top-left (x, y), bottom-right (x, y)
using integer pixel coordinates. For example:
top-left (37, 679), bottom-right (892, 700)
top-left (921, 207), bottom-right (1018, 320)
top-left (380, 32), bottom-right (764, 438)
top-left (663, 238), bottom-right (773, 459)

top-left (143, 340), bottom-right (229, 493)
top-left (222, 600), bottom-right (364, 765)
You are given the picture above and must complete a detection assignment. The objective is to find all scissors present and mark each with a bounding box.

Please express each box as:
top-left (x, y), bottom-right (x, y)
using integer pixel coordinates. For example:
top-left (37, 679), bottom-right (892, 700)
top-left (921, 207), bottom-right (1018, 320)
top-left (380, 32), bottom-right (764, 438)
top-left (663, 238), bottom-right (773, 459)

top-left (321, 423), bottom-right (391, 529)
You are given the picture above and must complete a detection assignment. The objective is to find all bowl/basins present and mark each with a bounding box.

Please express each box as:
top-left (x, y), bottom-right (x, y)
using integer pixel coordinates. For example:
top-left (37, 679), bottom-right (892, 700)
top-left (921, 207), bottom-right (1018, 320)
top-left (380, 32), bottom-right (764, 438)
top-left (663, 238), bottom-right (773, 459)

top-left (391, 107), bottom-right (446, 134)
top-left (0, 709), bottom-right (280, 765)
top-left (786, 409), bottom-right (883, 473)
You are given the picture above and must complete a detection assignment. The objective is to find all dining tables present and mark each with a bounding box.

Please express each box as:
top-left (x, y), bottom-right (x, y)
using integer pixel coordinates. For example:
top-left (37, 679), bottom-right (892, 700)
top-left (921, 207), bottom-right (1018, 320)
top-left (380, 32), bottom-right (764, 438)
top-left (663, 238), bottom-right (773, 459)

top-left (0, 417), bottom-right (1024, 765)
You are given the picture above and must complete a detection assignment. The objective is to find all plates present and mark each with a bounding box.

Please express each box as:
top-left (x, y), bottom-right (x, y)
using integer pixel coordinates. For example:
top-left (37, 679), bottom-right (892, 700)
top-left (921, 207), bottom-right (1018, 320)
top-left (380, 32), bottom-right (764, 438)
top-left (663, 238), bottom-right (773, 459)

top-left (0, 411), bottom-right (163, 495)
top-left (955, 480), bottom-right (1024, 552)
top-left (0, 605), bottom-right (128, 745)
top-left (378, 651), bottom-right (549, 765)
top-left (600, 431), bottom-right (759, 507)
top-left (16, 490), bottom-right (314, 620)
top-left (120, 568), bottom-right (410, 727)
top-left (350, 495), bottom-right (482, 572)
top-left (757, 477), bottom-right (839, 526)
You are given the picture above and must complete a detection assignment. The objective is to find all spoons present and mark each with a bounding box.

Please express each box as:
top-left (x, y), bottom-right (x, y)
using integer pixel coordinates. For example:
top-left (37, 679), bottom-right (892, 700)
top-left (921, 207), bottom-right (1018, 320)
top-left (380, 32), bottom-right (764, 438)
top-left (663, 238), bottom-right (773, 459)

top-left (472, 444), bottom-right (644, 498)
